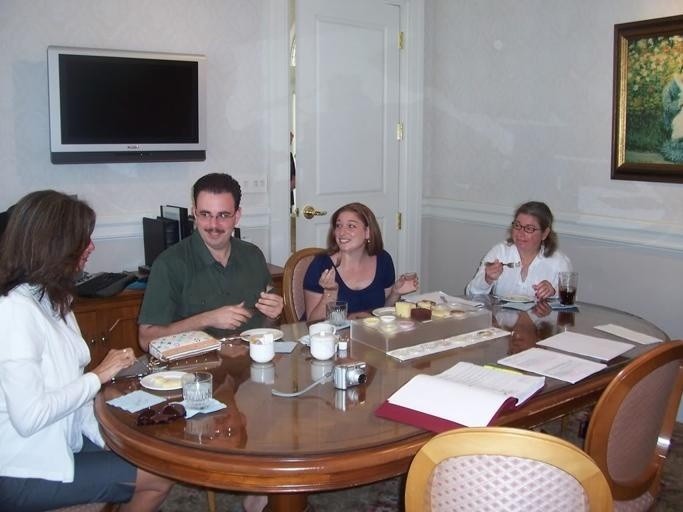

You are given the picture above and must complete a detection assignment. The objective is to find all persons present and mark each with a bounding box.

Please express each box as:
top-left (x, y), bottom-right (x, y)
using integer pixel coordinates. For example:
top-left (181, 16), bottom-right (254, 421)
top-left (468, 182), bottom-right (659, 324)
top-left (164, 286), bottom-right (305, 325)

top-left (464, 201), bottom-right (574, 300)
top-left (169, 331), bottom-right (286, 449)
top-left (138, 172), bottom-right (284, 353)
top-left (0, 190), bottom-right (174, 512)
top-left (300, 203), bottom-right (418, 324)
top-left (662, 66), bottom-right (682, 142)
top-left (492, 301), bottom-right (559, 356)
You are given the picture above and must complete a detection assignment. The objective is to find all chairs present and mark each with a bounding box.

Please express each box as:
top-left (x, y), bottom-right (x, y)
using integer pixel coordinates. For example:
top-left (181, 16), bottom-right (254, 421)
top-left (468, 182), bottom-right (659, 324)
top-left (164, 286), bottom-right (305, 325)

top-left (282, 247), bottom-right (327, 324)
top-left (402, 428), bottom-right (616, 512)
top-left (581, 340), bottom-right (682, 511)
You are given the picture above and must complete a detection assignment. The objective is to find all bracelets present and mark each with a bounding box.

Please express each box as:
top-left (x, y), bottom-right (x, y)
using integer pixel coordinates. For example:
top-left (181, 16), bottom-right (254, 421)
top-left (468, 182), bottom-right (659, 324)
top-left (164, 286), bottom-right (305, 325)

top-left (323, 292), bottom-right (337, 299)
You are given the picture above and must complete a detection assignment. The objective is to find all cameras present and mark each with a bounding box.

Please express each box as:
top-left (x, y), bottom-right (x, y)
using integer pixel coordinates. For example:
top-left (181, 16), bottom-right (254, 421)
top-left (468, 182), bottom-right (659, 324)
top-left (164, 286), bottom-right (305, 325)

top-left (334, 386), bottom-right (366, 412)
top-left (332, 360), bottom-right (368, 389)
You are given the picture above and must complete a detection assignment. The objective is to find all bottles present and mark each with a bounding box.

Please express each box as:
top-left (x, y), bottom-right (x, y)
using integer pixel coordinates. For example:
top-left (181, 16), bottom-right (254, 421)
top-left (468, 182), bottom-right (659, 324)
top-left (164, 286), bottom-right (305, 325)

top-left (338, 333), bottom-right (348, 350)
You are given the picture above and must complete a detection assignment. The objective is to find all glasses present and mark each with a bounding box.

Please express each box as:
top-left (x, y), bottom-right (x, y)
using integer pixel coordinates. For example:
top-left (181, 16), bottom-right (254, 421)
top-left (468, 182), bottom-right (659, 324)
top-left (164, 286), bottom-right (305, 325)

top-left (195, 210), bottom-right (236, 220)
top-left (137, 404), bottom-right (186, 426)
top-left (512, 222), bottom-right (542, 233)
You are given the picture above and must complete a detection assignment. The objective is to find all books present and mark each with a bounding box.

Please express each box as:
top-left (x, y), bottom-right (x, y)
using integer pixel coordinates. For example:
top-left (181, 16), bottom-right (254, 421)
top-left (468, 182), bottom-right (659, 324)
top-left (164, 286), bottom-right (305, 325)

top-left (374, 360), bottom-right (548, 434)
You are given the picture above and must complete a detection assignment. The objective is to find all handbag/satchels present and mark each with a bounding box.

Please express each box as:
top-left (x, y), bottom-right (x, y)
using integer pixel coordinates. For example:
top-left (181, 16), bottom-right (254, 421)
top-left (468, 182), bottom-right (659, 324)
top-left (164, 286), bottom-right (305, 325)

top-left (149, 331), bottom-right (222, 362)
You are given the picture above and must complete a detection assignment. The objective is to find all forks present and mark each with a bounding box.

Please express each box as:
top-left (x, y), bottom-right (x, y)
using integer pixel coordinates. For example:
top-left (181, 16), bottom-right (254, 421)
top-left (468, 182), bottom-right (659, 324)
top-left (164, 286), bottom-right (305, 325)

top-left (486, 261), bottom-right (520, 268)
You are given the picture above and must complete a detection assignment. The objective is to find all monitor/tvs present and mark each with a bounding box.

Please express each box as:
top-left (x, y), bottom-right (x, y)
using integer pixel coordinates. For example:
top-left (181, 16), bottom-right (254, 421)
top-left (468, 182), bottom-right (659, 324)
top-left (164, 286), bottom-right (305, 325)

top-left (47, 45), bottom-right (208, 159)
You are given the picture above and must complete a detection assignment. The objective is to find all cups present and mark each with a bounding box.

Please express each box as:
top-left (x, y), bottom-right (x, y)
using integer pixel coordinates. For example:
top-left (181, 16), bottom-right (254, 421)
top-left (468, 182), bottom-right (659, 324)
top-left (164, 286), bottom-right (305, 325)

top-left (309, 324), bottom-right (336, 336)
top-left (558, 272), bottom-right (578, 305)
top-left (184, 416), bottom-right (216, 443)
top-left (250, 334), bottom-right (275, 363)
top-left (181, 371), bottom-right (213, 408)
top-left (311, 361), bottom-right (334, 384)
top-left (557, 311), bottom-right (576, 328)
top-left (249, 362), bottom-right (276, 384)
top-left (325, 301), bottom-right (348, 326)
top-left (310, 332), bottom-right (335, 360)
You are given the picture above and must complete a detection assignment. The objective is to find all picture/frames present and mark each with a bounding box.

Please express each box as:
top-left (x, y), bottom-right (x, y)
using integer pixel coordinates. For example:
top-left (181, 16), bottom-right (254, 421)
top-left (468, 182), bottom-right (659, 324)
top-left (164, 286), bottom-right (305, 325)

top-left (610, 14), bottom-right (683, 183)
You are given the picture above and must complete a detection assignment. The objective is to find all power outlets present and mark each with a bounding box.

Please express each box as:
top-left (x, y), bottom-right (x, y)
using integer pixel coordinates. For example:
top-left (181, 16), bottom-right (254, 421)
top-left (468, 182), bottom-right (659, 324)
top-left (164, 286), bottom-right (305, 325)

top-left (238, 175), bottom-right (267, 194)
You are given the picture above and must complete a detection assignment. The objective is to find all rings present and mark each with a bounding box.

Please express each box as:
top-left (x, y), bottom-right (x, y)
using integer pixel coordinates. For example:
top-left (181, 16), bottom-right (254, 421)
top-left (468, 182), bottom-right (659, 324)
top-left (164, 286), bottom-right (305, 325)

top-left (122, 348), bottom-right (127, 352)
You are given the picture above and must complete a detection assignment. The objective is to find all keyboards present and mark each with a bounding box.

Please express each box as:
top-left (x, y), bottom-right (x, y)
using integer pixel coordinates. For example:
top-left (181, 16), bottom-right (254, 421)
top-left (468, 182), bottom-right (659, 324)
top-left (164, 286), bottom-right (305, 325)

top-left (77, 271), bottom-right (137, 299)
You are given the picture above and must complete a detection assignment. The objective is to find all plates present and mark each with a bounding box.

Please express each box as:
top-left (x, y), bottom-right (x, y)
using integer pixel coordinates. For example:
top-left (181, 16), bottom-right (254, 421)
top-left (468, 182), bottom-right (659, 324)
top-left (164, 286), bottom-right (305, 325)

top-left (372, 307), bottom-right (395, 316)
top-left (240, 328), bottom-right (284, 341)
top-left (140, 371), bottom-right (196, 391)
top-left (492, 294), bottom-right (534, 303)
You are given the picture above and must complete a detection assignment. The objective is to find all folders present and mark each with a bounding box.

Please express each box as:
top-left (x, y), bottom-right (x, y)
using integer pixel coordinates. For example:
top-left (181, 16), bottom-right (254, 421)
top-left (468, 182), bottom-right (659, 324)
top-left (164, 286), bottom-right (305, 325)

top-left (372, 377), bottom-right (548, 434)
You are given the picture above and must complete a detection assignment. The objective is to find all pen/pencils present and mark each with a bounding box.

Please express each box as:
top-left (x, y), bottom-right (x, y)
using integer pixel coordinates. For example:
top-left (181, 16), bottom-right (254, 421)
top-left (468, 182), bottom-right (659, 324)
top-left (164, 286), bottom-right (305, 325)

top-left (483, 364), bottom-right (523, 376)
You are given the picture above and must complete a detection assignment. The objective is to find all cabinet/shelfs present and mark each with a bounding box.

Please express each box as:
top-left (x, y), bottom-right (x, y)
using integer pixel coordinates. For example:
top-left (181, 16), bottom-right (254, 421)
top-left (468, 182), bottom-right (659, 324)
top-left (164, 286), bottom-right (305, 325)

top-left (71, 300), bottom-right (143, 379)
